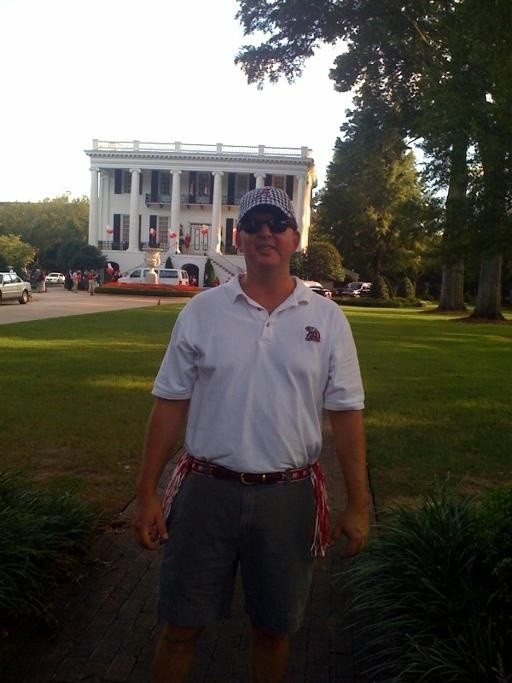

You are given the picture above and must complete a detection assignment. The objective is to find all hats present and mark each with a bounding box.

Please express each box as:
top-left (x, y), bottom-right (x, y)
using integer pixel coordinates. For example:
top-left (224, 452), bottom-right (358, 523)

top-left (235, 185), bottom-right (297, 228)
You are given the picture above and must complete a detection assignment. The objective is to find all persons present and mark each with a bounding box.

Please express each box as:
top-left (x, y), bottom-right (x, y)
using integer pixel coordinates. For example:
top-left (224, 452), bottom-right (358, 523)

top-left (64, 268), bottom-right (101, 296)
top-left (132, 183), bottom-right (372, 679)
top-left (112, 263), bottom-right (122, 281)
top-left (184, 232), bottom-right (191, 254)
top-left (39, 271), bottom-right (46, 292)
top-left (150, 224), bottom-right (156, 244)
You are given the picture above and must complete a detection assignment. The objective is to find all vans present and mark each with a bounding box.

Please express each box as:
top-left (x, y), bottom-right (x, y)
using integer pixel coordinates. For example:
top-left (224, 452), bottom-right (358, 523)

top-left (117, 267), bottom-right (189, 287)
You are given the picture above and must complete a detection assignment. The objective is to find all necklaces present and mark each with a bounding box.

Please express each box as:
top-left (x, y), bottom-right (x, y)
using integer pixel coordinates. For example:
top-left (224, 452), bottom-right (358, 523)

top-left (241, 279), bottom-right (287, 306)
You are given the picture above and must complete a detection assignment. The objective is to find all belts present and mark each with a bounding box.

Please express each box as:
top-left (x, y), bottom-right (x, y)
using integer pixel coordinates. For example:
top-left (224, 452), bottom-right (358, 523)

top-left (188, 459), bottom-right (318, 486)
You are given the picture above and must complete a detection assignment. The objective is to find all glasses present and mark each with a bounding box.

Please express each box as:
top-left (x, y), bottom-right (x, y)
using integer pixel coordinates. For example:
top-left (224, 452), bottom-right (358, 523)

top-left (241, 213), bottom-right (288, 233)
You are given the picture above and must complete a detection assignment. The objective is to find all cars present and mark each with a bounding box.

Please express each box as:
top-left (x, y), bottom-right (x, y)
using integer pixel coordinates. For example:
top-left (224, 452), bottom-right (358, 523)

top-left (0, 271), bottom-right (31, 303)
top-left (305, 280), bottom-right (333, 299)
top-left (333, 281), bottom-right (373, 297)
top-left (44, 272), bottom-right (65, 283)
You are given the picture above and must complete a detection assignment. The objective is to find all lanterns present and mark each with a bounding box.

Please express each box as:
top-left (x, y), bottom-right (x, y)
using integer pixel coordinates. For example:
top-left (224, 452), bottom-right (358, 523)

top-left (107, 227), bottom-right (113, 234)
top-left (168, 231), bottom-right (176, 238)
top-left (201, 227), bottom-right (208, 234)
top-left (107, 267), bottom-right (113, 274)
